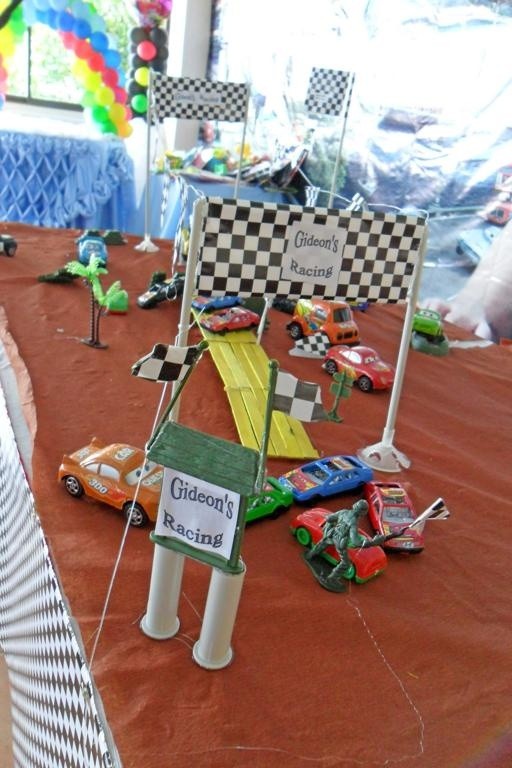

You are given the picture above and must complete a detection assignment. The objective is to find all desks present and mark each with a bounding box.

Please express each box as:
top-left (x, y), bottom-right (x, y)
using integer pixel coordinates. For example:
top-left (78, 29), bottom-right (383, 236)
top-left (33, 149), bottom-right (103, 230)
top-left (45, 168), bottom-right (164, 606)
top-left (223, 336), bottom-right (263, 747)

top-left (0, 222), bottom-right (512, 768)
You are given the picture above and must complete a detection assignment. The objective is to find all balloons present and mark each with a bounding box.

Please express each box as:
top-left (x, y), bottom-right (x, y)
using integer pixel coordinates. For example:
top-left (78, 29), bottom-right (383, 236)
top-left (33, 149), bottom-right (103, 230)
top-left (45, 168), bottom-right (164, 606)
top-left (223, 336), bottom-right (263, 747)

top-left (0, 0), bottom-right (175, 140)
